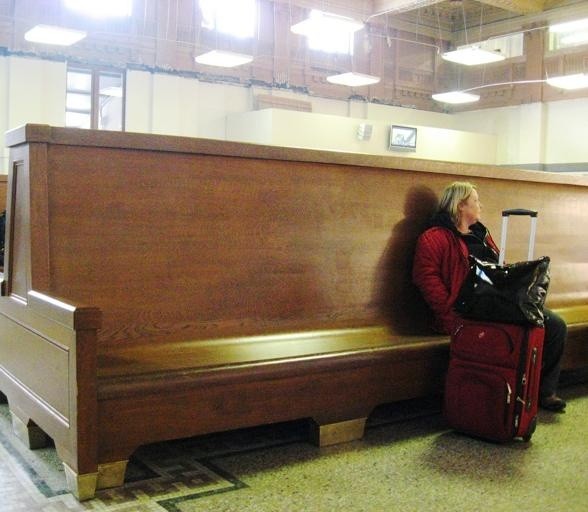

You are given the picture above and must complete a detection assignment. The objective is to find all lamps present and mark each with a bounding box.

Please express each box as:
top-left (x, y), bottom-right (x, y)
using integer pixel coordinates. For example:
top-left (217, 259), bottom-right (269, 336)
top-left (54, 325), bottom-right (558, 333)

top-left (26, 21), bottom-right (87, 46)
top-left (546, 71), bottom-right (588, 90)
top-left (431, 91), bottom-right (481, 103)
top-left (327, 72), bottom-right (380, 87)
top-left (441, 46), bottom-right (505, 65)
top-left (195, 49), bottom-right (253, 67)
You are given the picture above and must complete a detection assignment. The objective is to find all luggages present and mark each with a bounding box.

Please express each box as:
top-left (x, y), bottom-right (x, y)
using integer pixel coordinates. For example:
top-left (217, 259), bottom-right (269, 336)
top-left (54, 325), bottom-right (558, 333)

top-left (439, 206), bottom-right (550, 446)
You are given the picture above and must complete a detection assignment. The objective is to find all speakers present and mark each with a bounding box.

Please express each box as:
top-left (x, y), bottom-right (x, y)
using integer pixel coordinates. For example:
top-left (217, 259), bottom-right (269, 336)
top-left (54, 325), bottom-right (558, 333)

top-left (357, 122), bottom-right (373, 142)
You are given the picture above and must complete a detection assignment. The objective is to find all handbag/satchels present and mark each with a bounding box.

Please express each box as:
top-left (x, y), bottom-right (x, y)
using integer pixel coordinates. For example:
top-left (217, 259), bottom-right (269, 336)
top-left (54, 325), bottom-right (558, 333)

top-left (450, 252), bottom-right (551, 327)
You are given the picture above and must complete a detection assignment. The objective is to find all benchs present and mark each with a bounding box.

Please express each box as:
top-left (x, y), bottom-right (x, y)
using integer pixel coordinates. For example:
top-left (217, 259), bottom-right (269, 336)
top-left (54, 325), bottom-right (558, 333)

top-left (0, 122), bottom-right (588, 499)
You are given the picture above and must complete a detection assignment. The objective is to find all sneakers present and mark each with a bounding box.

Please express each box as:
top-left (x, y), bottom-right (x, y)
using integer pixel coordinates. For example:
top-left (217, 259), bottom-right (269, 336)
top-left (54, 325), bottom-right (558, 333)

top-left (537, 393), bottom-right (567, 412)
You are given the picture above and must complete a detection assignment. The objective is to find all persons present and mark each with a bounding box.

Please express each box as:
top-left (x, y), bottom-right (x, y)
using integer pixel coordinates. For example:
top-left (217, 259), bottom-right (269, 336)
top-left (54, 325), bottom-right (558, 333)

top-left (407, 181), bottom-right (567, 410)
